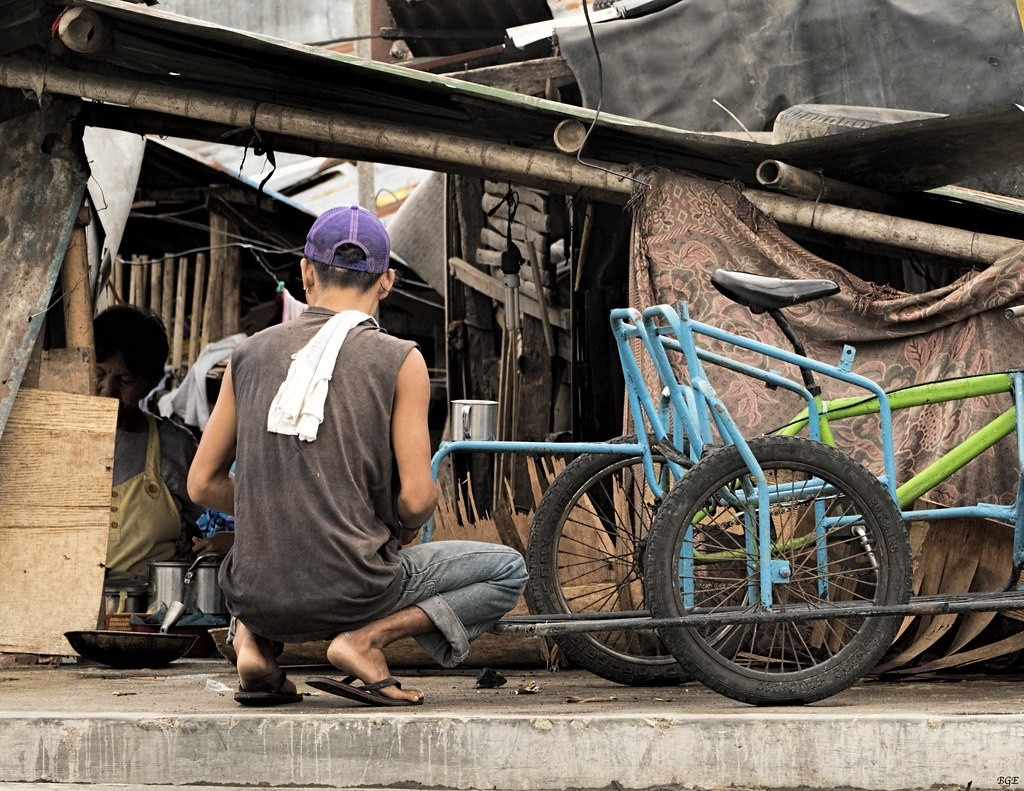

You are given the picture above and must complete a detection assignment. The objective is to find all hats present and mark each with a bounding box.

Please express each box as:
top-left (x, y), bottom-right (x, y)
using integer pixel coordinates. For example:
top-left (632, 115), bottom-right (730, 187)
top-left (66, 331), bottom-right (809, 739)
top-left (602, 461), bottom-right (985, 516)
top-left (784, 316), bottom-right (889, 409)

top-left (304, 204), bottom-right (390, 272)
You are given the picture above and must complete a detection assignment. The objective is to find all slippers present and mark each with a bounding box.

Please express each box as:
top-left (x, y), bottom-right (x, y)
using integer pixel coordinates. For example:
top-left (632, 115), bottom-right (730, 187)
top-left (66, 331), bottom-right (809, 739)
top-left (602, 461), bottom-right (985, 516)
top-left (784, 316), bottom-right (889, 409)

top-left (234, 670), bottom-right (303, 702)
top-left (305, 673), bottom-right (423, 705)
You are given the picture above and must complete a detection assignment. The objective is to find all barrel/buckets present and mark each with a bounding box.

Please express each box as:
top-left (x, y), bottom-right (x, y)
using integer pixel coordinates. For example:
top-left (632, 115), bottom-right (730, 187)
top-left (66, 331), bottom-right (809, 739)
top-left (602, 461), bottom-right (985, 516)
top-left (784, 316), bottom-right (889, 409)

top-left (145, 559), bottom-right (190, 609)
top-left (186, 554), bottom-right (224, 613)
top-left (107, 584), bottom-right (149, 612)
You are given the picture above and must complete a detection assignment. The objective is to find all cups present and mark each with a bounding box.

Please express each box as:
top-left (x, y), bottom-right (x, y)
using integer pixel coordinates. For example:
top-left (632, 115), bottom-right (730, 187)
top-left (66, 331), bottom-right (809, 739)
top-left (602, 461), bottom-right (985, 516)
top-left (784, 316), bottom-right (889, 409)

top-left (451, 400), bottom-right (500, 441)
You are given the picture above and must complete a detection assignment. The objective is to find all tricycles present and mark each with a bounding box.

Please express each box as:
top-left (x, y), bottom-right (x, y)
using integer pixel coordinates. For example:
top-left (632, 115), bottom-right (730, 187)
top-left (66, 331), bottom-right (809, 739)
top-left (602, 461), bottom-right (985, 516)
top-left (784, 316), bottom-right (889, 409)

top-left (415, 271), bottom-right (1022, 705)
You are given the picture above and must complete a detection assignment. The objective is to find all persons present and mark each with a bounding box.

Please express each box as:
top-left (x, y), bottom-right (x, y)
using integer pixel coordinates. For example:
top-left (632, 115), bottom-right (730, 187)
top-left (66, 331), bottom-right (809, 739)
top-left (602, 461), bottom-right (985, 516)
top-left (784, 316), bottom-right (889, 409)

top-left (92, 302), bottom-right (236, 584)
top-left (188, 206), bottom-right (527, 710)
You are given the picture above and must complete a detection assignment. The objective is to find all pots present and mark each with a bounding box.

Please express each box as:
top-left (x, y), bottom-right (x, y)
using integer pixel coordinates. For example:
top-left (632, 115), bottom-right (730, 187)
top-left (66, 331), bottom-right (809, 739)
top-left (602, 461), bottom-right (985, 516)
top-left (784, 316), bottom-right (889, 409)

top-left (63, 600), bottom-right (199, 668)
